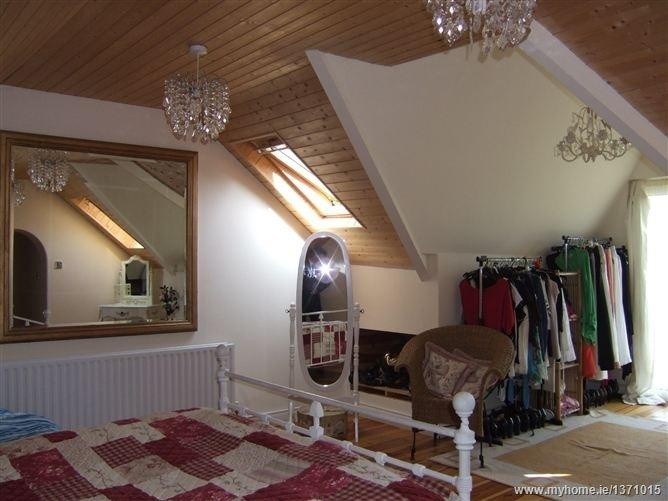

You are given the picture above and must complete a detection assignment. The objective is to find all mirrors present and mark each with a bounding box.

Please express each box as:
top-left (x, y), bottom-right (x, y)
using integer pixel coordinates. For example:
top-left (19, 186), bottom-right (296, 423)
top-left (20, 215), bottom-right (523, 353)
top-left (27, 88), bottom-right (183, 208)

top-left (296, 230), bottom-right (354, 392)
top-left (120, 254), bottom-right (150, 301)
top-left (1, 130), bottom-right (200, 345)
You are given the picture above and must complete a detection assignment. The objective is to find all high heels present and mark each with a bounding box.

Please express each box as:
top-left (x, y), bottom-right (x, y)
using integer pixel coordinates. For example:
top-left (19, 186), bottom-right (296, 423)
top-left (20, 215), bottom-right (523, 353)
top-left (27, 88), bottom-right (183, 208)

top-left (582, 378), bottom-right (618, 410)
top-left (482, 407), bottom-right (546, 439)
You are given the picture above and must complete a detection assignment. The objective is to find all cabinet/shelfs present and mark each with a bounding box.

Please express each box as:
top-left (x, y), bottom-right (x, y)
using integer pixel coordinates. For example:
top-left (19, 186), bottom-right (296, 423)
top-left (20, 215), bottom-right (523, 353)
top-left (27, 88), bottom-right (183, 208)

top-left (552, 271), bottom-right (582, 421)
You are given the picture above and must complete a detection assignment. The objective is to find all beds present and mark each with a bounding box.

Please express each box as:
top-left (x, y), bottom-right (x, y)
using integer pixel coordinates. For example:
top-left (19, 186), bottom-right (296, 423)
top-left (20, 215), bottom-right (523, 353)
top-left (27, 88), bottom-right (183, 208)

top-left (1, 345), bottom-right (476, 499)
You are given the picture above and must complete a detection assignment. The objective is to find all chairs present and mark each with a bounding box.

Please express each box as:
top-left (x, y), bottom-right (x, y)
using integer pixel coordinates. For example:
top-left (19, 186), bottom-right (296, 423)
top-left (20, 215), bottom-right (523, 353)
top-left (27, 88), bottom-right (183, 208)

top-left (383, 324), bottom-right (517, 467)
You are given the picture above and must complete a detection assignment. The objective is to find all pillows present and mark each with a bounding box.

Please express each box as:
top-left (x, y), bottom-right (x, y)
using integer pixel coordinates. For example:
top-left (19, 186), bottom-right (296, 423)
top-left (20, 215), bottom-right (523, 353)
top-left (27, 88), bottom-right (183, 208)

top-left (421, 341), bottom-right (492, 399)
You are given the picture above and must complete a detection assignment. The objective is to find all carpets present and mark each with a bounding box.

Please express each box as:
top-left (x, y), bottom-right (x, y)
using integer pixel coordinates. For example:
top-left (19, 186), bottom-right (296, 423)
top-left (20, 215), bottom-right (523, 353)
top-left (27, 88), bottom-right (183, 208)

top-left (427, 409), bottom-right (668, 501)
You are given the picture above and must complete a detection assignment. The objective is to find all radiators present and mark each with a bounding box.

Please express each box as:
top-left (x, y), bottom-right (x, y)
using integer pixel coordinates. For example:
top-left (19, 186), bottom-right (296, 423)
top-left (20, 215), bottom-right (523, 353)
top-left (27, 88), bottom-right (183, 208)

top-left (0, 342), bottom-right (237, 431)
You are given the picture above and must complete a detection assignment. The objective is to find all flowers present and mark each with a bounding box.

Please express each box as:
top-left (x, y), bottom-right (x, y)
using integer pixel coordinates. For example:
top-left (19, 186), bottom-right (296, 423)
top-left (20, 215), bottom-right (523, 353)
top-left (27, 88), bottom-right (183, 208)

top-left (159, 284), bottom-right (180, 319)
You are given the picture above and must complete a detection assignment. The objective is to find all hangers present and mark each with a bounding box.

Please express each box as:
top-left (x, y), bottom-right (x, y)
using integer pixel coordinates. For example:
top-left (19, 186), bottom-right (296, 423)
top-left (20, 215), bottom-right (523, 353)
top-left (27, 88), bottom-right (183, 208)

top-left (463, 255), bottom-right (544, 280)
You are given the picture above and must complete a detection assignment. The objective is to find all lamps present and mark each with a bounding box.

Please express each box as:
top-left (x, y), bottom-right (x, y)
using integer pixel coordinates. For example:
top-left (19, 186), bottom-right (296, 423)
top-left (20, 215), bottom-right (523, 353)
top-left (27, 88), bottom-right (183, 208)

top-left (162, 44), bottom-right (232, 145)
top-left (26, 147), bottom-right (72, 194)
top-left (423, 0), bottom-right (538, 57)
top-left (553, 106), bottom-right (633, 163)
